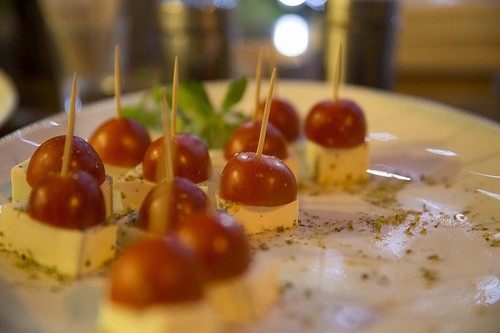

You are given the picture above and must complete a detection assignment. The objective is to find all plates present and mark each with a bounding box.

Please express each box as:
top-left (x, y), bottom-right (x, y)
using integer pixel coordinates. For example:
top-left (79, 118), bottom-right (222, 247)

top-left (0, 76), bottom-right (500, 331)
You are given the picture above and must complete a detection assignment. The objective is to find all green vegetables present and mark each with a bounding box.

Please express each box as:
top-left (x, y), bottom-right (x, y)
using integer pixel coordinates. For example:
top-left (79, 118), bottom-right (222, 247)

top-left (119, 74), bottom-right (256, 149)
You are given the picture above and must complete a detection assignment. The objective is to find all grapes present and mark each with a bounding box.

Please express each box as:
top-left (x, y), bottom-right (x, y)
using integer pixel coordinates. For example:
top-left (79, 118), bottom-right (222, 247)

top-left (27, 98), bottom-right (367, 306)
